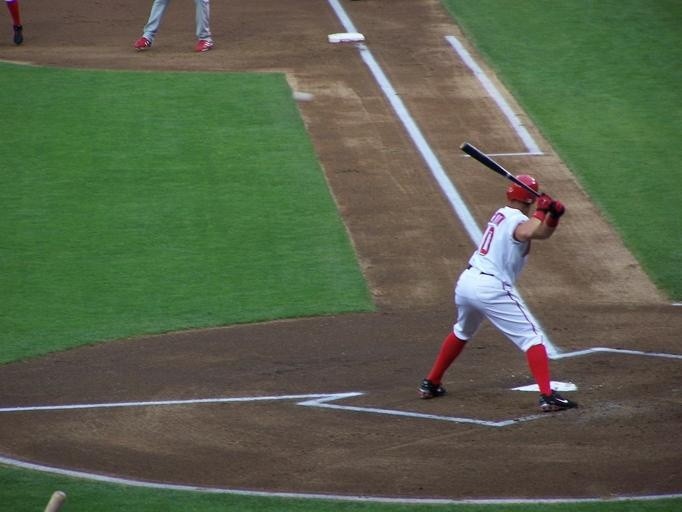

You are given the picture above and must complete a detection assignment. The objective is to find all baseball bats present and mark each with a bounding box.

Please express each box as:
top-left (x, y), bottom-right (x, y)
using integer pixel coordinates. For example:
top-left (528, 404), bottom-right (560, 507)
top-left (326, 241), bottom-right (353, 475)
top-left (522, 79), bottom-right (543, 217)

top-left (459, 141), bottom-right (540, 196)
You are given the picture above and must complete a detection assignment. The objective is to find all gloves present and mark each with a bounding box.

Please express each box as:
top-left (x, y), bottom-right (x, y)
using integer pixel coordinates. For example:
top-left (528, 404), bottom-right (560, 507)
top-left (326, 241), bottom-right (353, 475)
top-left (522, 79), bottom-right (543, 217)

top-left (532, 192), bottom-right (566, 227)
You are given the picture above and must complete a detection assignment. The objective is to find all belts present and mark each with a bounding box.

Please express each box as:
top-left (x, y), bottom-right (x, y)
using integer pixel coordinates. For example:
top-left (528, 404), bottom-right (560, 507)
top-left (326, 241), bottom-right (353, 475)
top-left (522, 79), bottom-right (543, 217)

top-left (468, 265), bottom-right (494, 276)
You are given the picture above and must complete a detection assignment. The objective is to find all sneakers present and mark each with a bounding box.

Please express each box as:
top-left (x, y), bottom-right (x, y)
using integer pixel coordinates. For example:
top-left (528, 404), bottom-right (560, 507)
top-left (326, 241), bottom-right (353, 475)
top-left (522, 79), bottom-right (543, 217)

top-left (194, 39), bottom-right (214, 52)
top-left (416, 380), bottom-right (446, 399)
top-left (13, 25), bottom-right (23, 44)
top-left (132, 38), bottom-right (152, 50)
top-left (539, 389), bottom-right (578, 412)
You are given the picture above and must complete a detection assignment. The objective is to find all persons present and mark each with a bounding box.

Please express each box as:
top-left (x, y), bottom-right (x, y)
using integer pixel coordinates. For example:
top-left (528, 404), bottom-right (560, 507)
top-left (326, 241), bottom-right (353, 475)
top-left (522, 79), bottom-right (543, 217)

top-left (419, 175), bottom-right (577, 413)
top-left (4, 0), bottom-right (24, 46)
top-left (132, 0), bottom-right (215, 52)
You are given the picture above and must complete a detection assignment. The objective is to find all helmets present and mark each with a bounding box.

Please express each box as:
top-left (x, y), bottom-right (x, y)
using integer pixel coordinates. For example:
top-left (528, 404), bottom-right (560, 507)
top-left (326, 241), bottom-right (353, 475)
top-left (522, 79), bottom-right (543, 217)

top-left (506, 174), bottom-right (539, 204)
